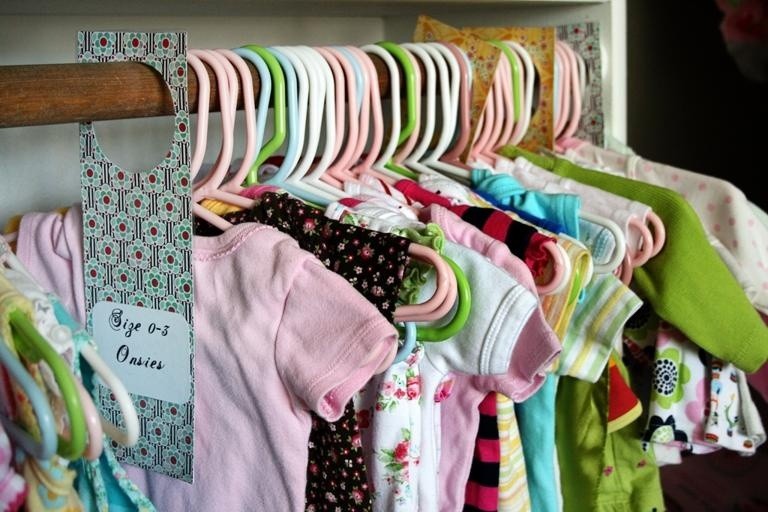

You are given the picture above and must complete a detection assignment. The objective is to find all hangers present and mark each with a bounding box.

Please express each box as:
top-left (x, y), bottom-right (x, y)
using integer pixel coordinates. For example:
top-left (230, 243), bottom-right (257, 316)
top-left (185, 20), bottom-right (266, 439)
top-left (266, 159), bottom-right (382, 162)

top-left (0, 235), bottom-right (143, 463)
